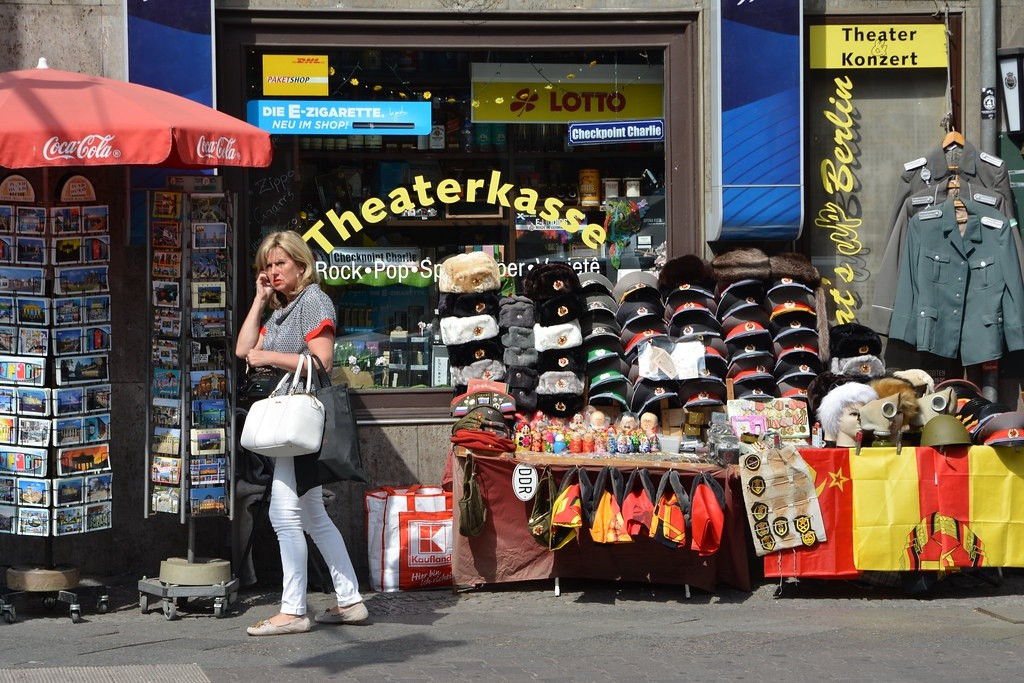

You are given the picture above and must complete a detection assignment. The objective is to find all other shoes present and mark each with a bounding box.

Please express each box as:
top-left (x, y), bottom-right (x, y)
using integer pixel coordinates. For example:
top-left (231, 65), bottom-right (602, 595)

top-left (247, 615), bottom-right (312, 636)
top-left (314, 603), bottom-right (369, 624)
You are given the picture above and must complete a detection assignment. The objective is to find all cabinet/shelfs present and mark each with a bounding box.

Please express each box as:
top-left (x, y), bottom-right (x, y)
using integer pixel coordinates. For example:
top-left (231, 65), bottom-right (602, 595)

top-left (244, 111), bottom-right (671, 406)
top-left (137, 174), bottom-right (239, 619)
top-left (0, 215), bottom-right (113, 621)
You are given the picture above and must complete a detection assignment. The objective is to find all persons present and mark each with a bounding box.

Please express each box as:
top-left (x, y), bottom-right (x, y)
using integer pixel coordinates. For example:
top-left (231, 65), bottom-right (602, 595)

top-left (818, 377), bottom-right (919, 449)
top-left (234, 229), bottom-right (369, 636)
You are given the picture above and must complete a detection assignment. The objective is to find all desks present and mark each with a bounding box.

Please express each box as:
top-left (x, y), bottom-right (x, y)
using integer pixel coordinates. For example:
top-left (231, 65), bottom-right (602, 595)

top-left (453, 446), bottom-right (740, 598)
top-left (740, 443), bottom-right (1024, 590)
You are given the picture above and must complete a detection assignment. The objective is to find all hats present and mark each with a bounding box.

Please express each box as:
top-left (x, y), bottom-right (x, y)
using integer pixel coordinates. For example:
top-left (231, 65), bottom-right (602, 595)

top-left (439, 251), bottom-right (1024, 452)
top-left (527, 464), bottom-right (725, 556)
top-left (458, 454), bottom-right (486, 537)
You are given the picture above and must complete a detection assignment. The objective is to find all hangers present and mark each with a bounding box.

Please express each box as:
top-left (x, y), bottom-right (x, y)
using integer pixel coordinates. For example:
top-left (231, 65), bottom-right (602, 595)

top-left (945, 175), bottom-right (962, 188)
top-left (952, 200), bottom-right (969, 224)
top-left (941, 111), bottom-right (964, 169)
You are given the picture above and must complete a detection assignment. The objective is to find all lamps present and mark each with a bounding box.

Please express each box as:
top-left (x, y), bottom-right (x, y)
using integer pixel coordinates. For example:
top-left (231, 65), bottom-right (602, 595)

top-left (998, 48), bottom-right (1023, 163)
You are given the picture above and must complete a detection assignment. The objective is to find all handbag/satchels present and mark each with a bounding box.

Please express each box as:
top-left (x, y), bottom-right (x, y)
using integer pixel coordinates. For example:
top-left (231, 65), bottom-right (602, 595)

top-left (240, 354), bottom-right (325, 457)
top-left (294, 355), bottom-right (368, 497)
top-left (365, 483), bottom-right (453, 593)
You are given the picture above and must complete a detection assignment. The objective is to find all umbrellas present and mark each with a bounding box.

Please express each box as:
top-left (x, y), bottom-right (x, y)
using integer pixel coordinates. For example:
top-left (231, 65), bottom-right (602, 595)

top-left (0, 56), bottom-right (272, 624)
